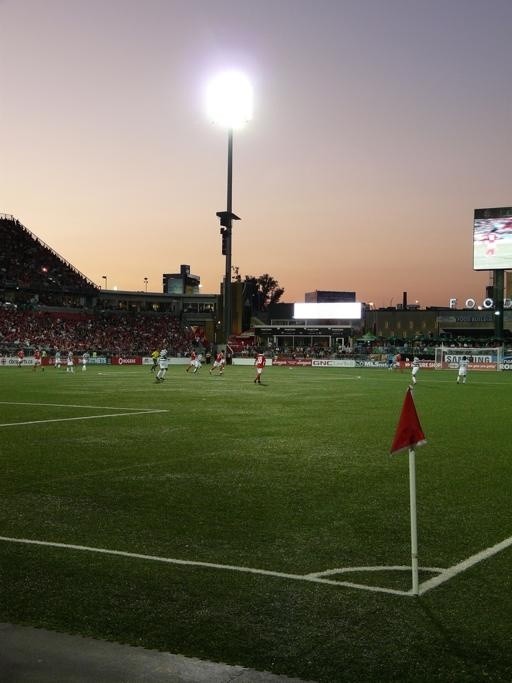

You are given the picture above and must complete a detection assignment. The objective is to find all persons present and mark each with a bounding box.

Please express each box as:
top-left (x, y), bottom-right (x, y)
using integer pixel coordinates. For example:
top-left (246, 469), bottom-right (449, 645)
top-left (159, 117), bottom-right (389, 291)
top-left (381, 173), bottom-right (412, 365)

top-left (34, 348), bottom-right (40, 368)
top-left (209, 349), bottom-right (225, 374)
top-left (150, 347), bottom-right (159, 371)
top-left (194, 351), bottom-right (204, 373)
top-left (483, 227), bottom-right (500, 256)
top-left (253, 350), bottom-right (266, 382)
top-left (55, 351), bottom-right (62, 368)
top-left (410, 355), bottom-right (419, 385)
top-left (1, 215), bottom-right (213, 360)
top-left (241, 334), bottom-right (504, 360)
top-left (18, 348), bottom-right (25, 367)
top-left (457, 355), bottom-right (468, 384)
top-left (387, 352), bottom-right (395, 372)
top-left (156, 345), bottom-right (169, 380)
top-left (185, 348), bottom-right (196, 372)
top-left (82, 355), bottom-right (87, 370)
top-left (40, 351), bottom-right (49, 375)
top-left (67, 350), bottom-right (74, 372)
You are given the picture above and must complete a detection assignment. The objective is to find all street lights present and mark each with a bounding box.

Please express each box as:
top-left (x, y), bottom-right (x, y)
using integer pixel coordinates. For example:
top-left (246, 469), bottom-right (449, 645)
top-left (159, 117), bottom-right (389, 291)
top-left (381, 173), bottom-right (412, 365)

top-left (216, 71), bottom-right (240, 343)
